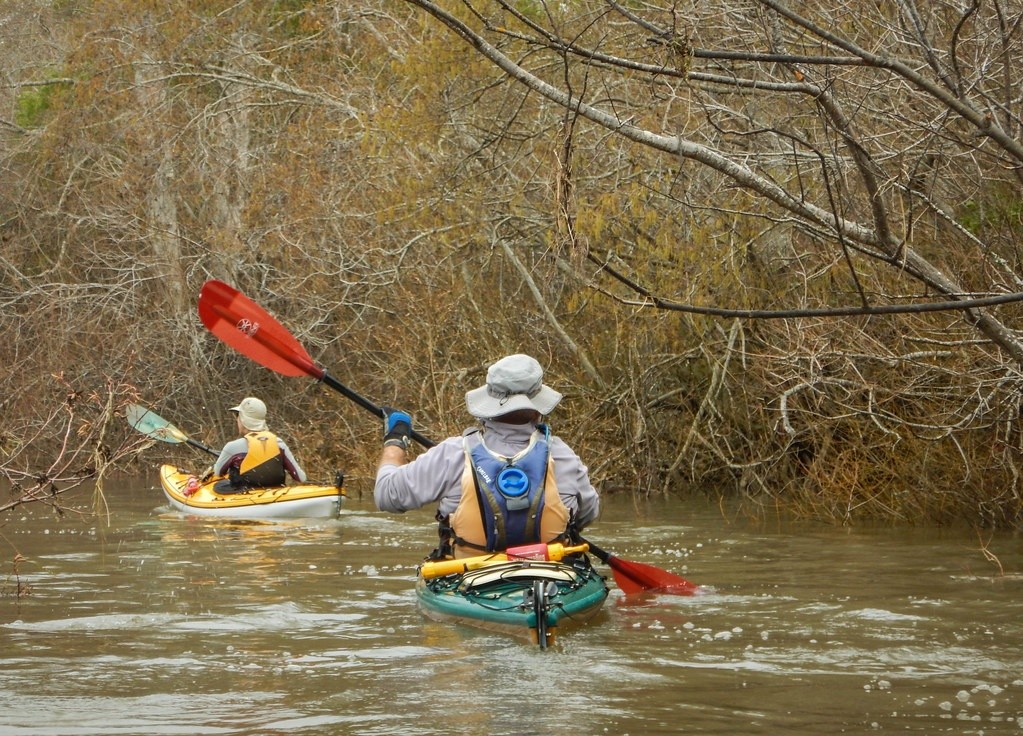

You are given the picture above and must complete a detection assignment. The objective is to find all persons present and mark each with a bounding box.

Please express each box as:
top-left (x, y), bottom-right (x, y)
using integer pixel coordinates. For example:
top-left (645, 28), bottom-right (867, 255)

top-left (375, 353), bottom-right (599, 560)
top-left (212, 397), bottom-right (307, 492)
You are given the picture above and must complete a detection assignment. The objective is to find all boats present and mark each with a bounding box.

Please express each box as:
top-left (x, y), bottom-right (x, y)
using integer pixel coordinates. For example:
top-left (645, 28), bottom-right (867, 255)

top-left (413, 531), bottom-right (611, 652)
top-left (160, 463), bottom-right (348, 519)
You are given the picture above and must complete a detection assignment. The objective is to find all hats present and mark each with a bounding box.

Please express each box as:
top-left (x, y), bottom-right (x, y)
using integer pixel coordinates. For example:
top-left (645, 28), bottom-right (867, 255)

top-left (228, 397), bottom-right (267, 431)
top-left (465, 354), bottom-right (564, 418)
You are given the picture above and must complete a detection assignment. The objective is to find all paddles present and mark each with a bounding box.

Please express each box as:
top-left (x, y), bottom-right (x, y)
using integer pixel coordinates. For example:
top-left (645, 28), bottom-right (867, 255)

top-left (124, 402), bottom-right (219, 458)
top-left (206, 281), bottom-right (699, 601)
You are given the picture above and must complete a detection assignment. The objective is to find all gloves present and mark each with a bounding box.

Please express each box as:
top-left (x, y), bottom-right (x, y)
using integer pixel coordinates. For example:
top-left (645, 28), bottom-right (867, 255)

top-left (380, 405), bottom-right (411, 451)
